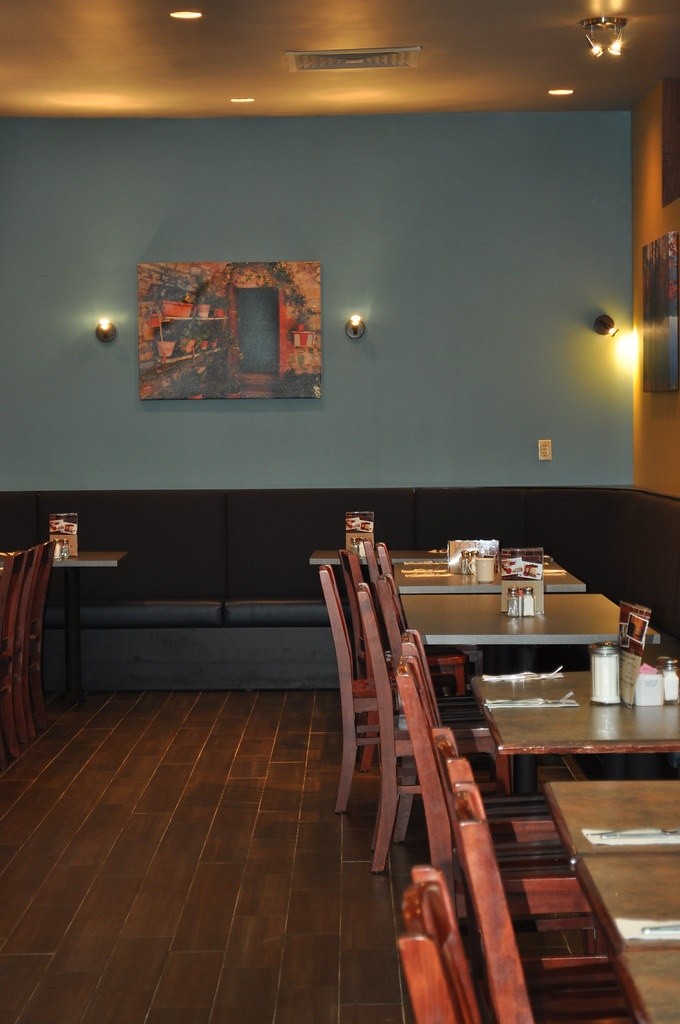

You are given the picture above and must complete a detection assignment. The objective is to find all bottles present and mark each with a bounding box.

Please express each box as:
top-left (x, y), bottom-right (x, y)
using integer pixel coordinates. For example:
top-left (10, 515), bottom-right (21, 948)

top-left (505, 587), bottom-right (536, 618)
top-left (471, 551), bottom-right (479, 574)
top-left (359, 536), bottom-right (366, 557)
top-left (351, 538), bottom-right (359, 552)
top-left (61, 538), bottom-right (69, 559)
top-left (460, 551), bottom-right (470, 575)
top-left (53, 538), bottom-right (61, 559)
top-left (656, 655), bottom-right (680, 702)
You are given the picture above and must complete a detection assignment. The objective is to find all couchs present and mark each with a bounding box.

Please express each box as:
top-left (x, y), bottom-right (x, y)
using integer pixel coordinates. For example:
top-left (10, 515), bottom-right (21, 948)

top-left (0, 485), bottom-right (680, 780)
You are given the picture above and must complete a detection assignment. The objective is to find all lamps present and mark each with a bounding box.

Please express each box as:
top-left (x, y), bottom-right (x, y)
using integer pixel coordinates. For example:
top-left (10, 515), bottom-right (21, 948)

top-left (594, 315), bottom-right (621, 339)
top-left (95, 319), bottom-right (116, 343)
top-left (607, 24), bottom-right (624, 56)
top-left (345, 315), bottom-right (366, 340)
top-left (584, 25), bottom-right (603, 57)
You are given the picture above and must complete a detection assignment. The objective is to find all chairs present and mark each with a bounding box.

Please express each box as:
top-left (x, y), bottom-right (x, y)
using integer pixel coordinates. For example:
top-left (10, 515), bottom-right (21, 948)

top-left (316, 539), bottom-right (641, 1024)
top-left (0, 539), bottom-right (56, 770)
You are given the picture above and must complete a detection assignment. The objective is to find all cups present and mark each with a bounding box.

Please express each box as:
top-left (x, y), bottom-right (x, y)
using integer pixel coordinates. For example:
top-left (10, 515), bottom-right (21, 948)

top-left (469, 555), bottom-right (497, 584)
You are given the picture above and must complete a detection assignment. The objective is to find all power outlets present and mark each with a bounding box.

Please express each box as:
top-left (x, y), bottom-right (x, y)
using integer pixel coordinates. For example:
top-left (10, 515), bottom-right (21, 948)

top-left (538, 440), bottom-right (552, 461)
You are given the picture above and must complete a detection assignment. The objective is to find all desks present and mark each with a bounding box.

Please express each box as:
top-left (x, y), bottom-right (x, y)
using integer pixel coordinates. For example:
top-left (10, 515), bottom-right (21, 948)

top-left (52, 551), bottom-right (129, 701)
top-left (544, 779), bottom-right (680, 861)
top-left (309, 550), bottom-right (504, 683)
top-left (392, 561), bottom-right (585, 673)
top-left (400, 593), bottom-right (661, 674)
top-left (470, 671), bottom-right (680, 797)
top-left (574, 852), bottom-right (680, 958)
top-left (612, 949), bottom-right (680, 1024)
